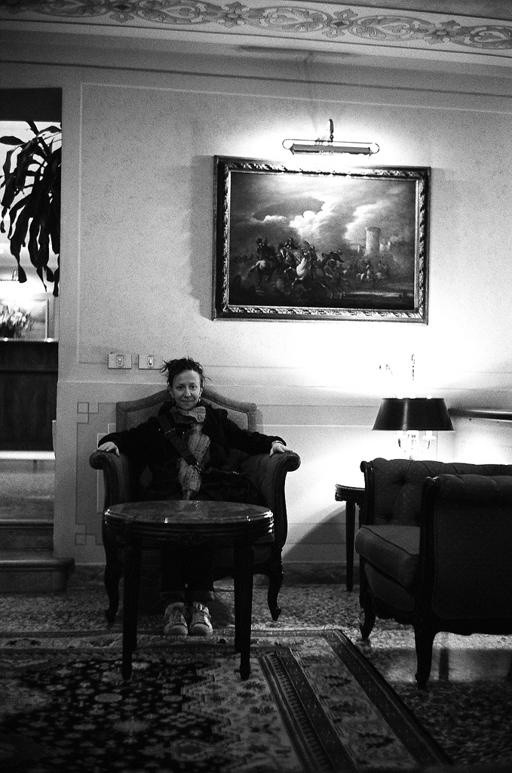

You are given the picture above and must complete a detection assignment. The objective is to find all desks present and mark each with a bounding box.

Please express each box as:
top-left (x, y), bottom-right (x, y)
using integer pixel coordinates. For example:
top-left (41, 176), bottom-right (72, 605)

top-left (335, 485), bottom-right (365, 592)
top-left (102, 500), bottom-right (276, 680)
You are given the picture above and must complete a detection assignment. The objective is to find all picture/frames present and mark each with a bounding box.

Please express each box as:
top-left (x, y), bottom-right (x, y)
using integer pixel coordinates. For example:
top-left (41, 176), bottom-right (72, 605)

top-left (212, 155), bottom-right (430, 324)
top-left (0, 298), bottom-right (49, 340)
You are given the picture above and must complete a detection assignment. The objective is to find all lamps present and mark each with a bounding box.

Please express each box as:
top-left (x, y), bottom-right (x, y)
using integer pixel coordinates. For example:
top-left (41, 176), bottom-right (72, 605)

top-left (0, 269), bottom-right (20, 281)
top-left (372, 398), bottom-right (455, 460)
top-left (282, 119), bottom-right (380, 156)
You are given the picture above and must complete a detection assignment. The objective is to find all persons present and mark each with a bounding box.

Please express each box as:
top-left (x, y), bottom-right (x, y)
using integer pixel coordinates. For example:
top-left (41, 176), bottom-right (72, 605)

top-left (256, 236), bottom-right (393, 284)
top-left (96, 356), bottom-right (294, 637)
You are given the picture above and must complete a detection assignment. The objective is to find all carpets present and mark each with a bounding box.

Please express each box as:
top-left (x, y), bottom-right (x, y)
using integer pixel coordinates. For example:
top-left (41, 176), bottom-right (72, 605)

top-left (0, 629), bottom-right (463, 773)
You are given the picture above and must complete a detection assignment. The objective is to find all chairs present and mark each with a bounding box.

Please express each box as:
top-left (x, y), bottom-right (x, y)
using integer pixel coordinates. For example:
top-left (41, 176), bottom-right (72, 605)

top-left (355, 458), bottom-right (512, 689)
top-left (89, 388), bottom-right (300, 620)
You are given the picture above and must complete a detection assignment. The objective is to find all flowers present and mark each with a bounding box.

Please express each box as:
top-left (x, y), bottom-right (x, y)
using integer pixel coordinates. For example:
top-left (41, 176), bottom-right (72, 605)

top-left (0, 304), bottom-right (34, 339)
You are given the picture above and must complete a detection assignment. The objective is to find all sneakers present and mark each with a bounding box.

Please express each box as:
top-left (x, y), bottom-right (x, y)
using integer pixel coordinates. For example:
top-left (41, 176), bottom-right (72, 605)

top-left (165, 602), bottom-right (214, 635)
